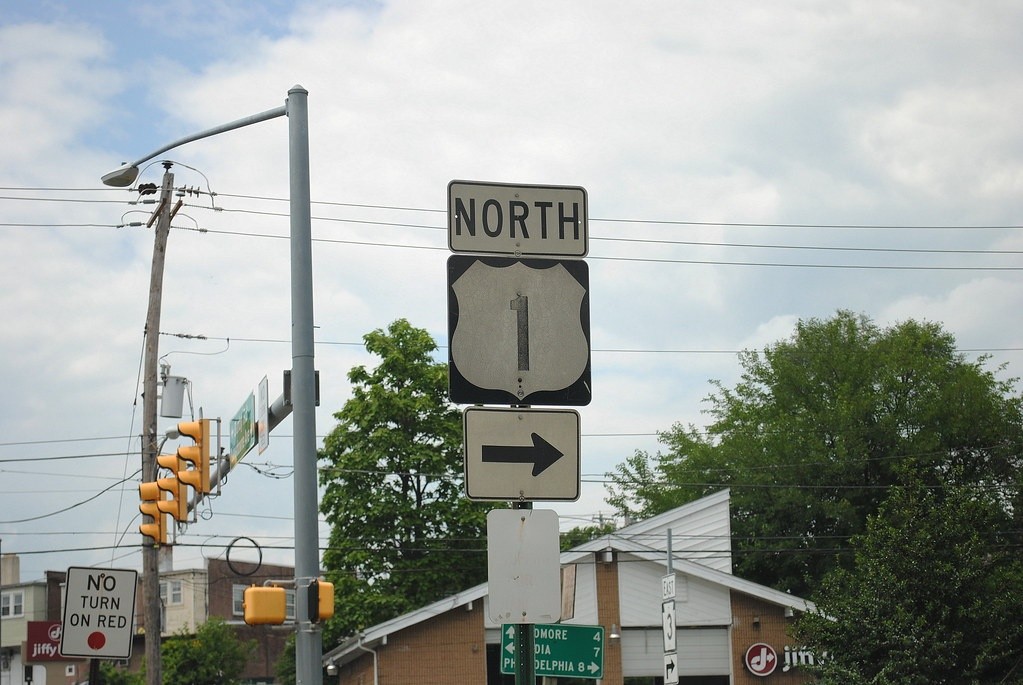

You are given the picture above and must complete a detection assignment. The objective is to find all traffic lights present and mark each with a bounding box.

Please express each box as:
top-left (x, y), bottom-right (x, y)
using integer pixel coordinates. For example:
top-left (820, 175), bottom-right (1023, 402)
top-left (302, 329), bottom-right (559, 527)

top-left (155, 453), bottom-right (188, 524)
top-left (137, 477), bottom-right (170, 547)
top-left (307, 579), bottom-right (335, 624)
top-left (178, 417), bottom-right (213, 494)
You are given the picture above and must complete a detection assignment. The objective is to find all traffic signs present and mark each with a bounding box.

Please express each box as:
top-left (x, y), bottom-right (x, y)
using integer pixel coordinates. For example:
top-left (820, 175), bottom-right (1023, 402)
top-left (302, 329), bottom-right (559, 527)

top-left (500, 622), bottom-right (605, 679)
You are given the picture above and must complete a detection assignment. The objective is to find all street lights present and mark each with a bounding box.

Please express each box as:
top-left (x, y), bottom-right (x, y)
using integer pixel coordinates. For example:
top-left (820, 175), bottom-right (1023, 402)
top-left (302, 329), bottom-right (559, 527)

top-left (141, 426), bottom-right (187, 685)
top-left (95, 81), bottom-right (325, 685)
